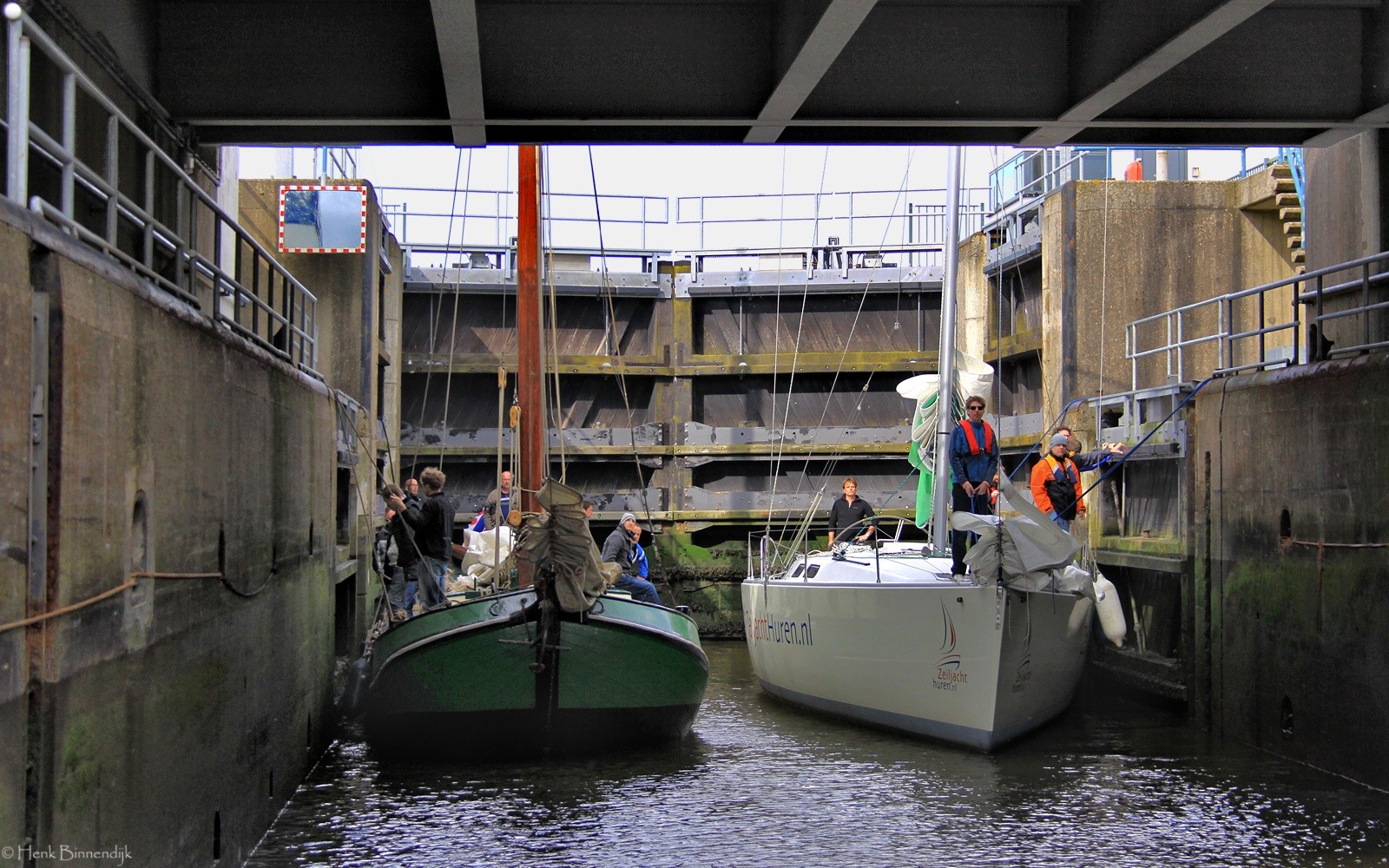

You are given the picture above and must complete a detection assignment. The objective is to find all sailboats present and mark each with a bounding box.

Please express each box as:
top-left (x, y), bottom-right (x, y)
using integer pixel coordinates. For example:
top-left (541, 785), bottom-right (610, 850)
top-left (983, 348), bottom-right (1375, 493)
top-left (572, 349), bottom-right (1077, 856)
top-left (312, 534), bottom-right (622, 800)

top-left (742, 146), bottom-right (1105, 753)
top-left (348, 146), bottom-right (727, 763)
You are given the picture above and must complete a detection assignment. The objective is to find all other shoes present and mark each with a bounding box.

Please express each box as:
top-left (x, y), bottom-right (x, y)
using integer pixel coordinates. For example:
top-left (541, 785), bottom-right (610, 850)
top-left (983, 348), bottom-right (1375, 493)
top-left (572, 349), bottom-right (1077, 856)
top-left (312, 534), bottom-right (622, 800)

top-left (953, 573), bottom-right (964, 581)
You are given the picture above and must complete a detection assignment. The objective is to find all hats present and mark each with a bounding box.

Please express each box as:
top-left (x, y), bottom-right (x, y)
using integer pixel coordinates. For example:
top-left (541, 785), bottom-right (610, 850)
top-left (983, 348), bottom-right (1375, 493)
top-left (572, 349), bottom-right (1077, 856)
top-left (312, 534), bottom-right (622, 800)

top-left (621, 513), bottom-right (636, 524)
top-left (1050, 433), bottom-right (1069, 449)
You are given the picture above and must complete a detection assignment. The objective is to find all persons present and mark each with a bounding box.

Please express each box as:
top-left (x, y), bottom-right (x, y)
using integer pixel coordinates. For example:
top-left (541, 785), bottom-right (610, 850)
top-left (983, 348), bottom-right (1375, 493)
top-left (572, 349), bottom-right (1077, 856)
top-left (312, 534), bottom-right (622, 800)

top-left (381, 466), bottom-right (662, 622)
top-left (949, 396), bottom-right (998, 578)
top-left (988, 464), bottom-right (1005, 515)
top-left (1030, 426), bottom-right (1125, 533)
top-left (827, 477), bottom-right (880, 551)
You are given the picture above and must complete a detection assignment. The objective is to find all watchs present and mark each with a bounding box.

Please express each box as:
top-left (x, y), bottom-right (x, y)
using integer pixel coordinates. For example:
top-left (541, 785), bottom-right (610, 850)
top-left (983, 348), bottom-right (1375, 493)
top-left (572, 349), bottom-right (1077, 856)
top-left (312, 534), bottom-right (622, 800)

top-left (865, 533), bottom-right (870, 537)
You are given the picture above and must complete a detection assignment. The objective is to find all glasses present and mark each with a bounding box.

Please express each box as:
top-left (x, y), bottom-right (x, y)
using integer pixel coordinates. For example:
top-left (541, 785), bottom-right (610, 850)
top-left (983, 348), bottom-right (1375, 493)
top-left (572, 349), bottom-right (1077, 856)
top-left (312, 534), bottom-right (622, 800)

top-left (968, 406), bottom-right (984, 410)
